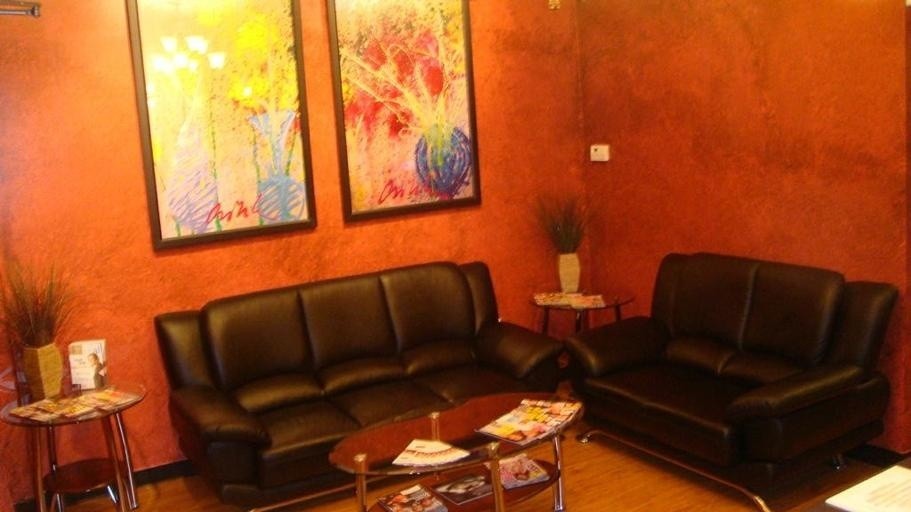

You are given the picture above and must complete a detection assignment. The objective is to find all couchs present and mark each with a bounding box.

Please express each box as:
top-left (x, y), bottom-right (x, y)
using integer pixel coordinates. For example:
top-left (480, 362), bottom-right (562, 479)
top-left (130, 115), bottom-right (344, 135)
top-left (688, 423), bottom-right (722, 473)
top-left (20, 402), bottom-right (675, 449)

top-left (564, 253), bottom-right (899, 512)
top-left (155, 261), bottom-right (574, 512)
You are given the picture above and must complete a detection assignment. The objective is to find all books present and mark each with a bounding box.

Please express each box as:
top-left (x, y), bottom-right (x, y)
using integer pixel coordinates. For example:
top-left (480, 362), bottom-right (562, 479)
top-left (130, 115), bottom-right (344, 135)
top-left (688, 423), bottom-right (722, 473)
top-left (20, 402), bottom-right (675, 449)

top-left (534, 291), bottom-right (605, 309)
top-left (483, 453), bottom-right (550, 490)
top-left (431, 474), bottom-right (493, 505)
top-left (9, 339), bottom-right (135, 422)
top-left (376, 483), bottom-right (449, 512)
top-left (479, 398), bottom-right (583, 446)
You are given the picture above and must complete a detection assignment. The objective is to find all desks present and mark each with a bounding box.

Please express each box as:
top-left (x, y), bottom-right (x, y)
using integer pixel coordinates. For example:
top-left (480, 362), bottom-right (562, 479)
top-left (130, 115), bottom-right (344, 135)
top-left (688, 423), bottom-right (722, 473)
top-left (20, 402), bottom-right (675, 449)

top-left (528, 289), bottom-right (633, 334)
top-left (0, 381), bottom-right (146, 512)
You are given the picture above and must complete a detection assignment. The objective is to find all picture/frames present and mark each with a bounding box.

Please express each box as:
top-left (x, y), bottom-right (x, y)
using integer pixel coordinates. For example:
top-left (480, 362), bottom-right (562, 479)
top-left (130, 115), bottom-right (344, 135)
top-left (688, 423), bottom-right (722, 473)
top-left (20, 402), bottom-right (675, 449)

top-left (126, 1), bottom-right (316, 250)
top-left (325, 0), bottom-right (481, 222)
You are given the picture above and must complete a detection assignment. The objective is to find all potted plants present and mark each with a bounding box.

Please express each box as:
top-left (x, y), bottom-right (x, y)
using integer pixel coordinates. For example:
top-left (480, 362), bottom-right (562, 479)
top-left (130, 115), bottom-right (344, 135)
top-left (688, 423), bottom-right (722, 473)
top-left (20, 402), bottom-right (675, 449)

top-left (533, 194), bottom-right (590, 293)
top-left (0, 262), bottom-right (74, 399)
